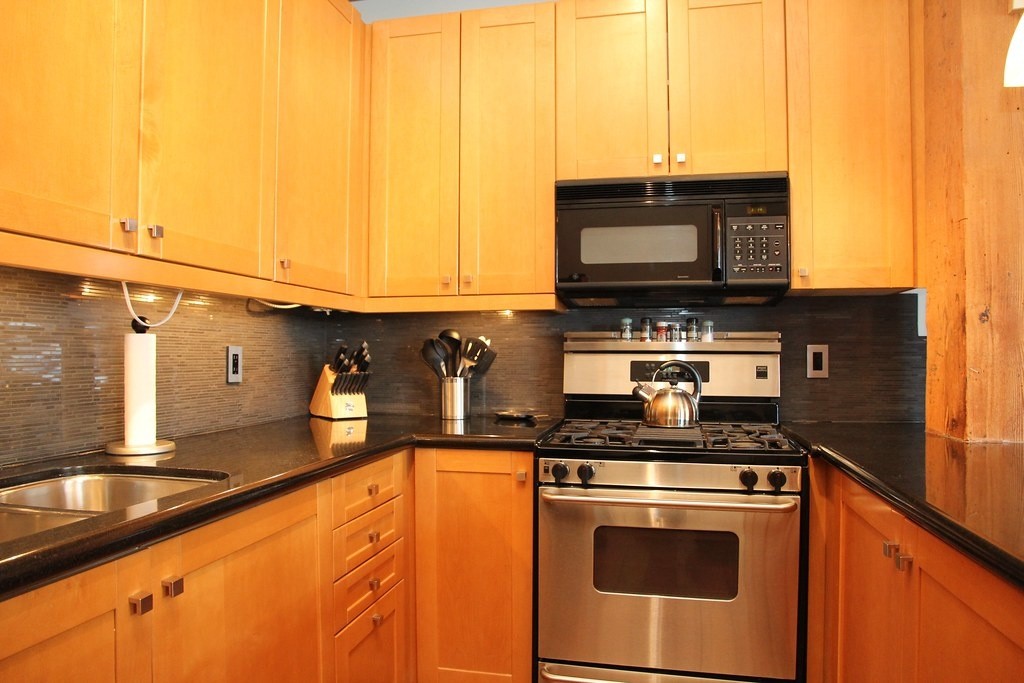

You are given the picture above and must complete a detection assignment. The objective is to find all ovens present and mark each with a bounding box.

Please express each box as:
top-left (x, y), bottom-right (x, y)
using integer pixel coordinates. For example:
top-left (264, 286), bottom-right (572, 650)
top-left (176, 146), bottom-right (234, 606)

top-left (533, 440), bottom-right (808, 683)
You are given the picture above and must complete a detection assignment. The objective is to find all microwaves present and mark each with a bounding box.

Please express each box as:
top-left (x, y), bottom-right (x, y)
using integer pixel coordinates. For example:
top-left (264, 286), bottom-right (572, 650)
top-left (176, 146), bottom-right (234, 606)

top-left (553, 170), bottom-right (791, 308)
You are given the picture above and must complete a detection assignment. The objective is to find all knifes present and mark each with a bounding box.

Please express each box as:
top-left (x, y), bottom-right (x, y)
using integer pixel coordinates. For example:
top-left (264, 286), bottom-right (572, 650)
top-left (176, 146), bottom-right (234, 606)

top-left (332, 341), bottom-right (373, 394)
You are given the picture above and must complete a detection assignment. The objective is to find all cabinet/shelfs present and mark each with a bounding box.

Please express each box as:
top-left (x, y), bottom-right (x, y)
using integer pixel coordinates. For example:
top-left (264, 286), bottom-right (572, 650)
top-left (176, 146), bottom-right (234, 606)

top-left (826, 464), bottom-right (1024, 683)
top-left (0, 0), bottom-right (925, 313)
top-left (0, 448), bottom-right (532, 682)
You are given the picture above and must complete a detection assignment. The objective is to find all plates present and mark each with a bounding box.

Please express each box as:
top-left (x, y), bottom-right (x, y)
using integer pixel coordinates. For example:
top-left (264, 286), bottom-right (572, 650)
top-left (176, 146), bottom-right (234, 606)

top-left (495, 411), bottom-right (535, 419)
top-left (494, 420), bottom-right (536, 428)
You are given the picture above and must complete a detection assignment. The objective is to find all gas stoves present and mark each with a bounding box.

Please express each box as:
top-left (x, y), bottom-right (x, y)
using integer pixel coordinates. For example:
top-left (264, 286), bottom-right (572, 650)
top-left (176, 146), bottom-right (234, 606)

top-left (535, 330), bottom-right (808, 467)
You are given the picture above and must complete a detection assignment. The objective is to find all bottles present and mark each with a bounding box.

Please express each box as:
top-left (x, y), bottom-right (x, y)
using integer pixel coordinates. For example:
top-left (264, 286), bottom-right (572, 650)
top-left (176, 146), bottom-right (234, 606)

top-left (656, 321), bottom-right (668, 341)
top-left (686, 317), bottom-right (699, 338)
top-left (670, 324), bottom-right (681, 341)
top-left (621, 317), bottom-right (632, 339)
top-left (701, 320), bottom-right (714, 342)
top-left (640, 317), bottom-right (653, 341)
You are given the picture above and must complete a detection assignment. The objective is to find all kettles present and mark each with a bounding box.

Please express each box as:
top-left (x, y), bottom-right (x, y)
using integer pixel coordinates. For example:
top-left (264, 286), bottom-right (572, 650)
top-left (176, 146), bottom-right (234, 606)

top-left (633, 360), bottom-right (703, 428)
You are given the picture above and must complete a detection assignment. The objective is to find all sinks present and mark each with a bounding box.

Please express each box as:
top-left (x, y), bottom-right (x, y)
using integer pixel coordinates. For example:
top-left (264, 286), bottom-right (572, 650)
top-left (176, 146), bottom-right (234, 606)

top-left (0, 504), bottom-right (106, 543)
top-left (0, 473), bottom-right (224, 514)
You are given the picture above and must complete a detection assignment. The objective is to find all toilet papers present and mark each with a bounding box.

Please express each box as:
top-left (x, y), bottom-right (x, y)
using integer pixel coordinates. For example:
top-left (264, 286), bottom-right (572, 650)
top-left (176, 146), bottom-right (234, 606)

top-left (123, 333), bottom-right (157, 446)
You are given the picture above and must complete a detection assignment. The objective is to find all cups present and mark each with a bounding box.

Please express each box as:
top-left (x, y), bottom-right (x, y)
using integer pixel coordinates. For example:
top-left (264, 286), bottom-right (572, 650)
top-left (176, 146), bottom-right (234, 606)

top-left (442, 419), bottom-right (464, 435)
top-left (440, 377), bottom-right (465, 420)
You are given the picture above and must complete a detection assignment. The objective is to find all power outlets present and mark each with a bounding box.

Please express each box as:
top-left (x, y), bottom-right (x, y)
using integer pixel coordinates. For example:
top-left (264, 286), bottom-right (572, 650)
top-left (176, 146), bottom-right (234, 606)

top-left (227, 345), bottom-right (244, 384)
top-left (806, 344), bottom-right (829, 378)
